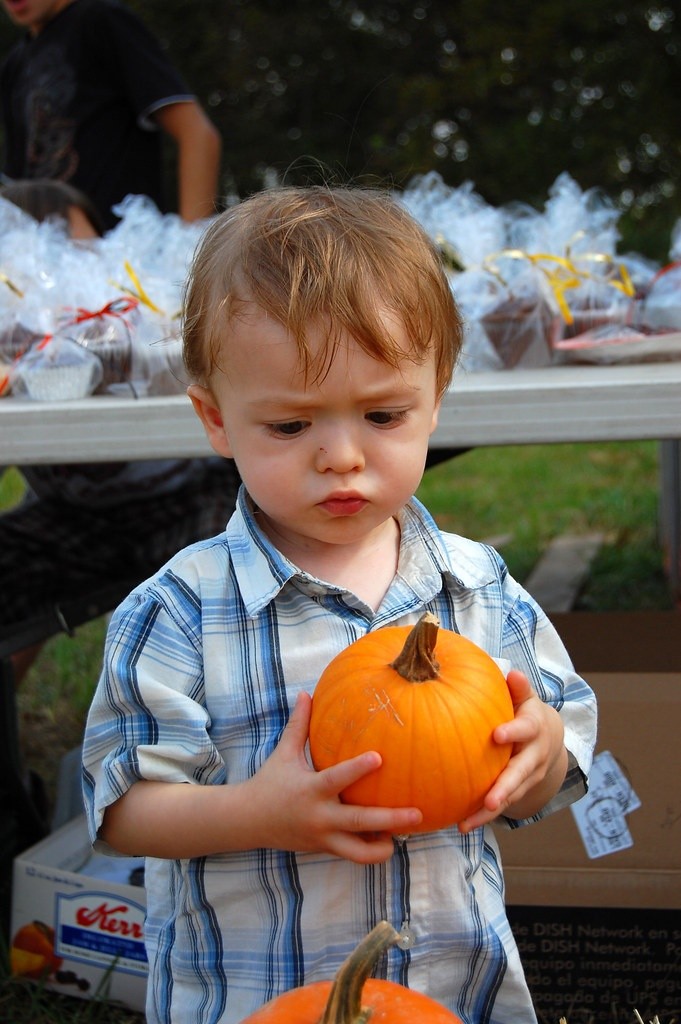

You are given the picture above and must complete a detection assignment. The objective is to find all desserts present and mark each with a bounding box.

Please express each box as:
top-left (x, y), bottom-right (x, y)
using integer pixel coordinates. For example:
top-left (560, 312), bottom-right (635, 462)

top-left (0, 258), bottom-right (681, 403)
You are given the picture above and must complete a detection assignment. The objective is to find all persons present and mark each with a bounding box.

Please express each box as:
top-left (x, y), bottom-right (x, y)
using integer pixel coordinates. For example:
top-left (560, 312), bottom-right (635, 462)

top-left (82, 188), bottom-right (597, 1024)
top-left (0, 0), bottom-right (224, 239)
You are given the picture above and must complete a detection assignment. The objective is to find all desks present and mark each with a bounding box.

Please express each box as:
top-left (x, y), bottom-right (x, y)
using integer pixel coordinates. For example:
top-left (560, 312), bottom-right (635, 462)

top-left (0, 371), bottom-right (681, 601)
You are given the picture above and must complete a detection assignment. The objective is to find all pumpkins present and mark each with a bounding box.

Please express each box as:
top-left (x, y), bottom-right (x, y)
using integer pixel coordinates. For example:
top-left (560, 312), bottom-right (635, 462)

top-left (309, 610), bottom-right (515, 835)
top-left (237, 920), bottom-right (464, 1024)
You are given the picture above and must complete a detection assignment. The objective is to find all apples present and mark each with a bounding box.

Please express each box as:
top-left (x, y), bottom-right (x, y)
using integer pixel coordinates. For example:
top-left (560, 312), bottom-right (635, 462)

top-left (11, 920), bottom-right (63, 979)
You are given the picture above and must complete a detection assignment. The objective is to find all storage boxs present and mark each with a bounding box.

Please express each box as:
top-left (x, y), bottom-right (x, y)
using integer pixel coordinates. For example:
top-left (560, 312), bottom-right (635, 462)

top-left (485, 611), bottom-right (681, 1024)
top-left (12, 812), bottom-right (147, 1019)
